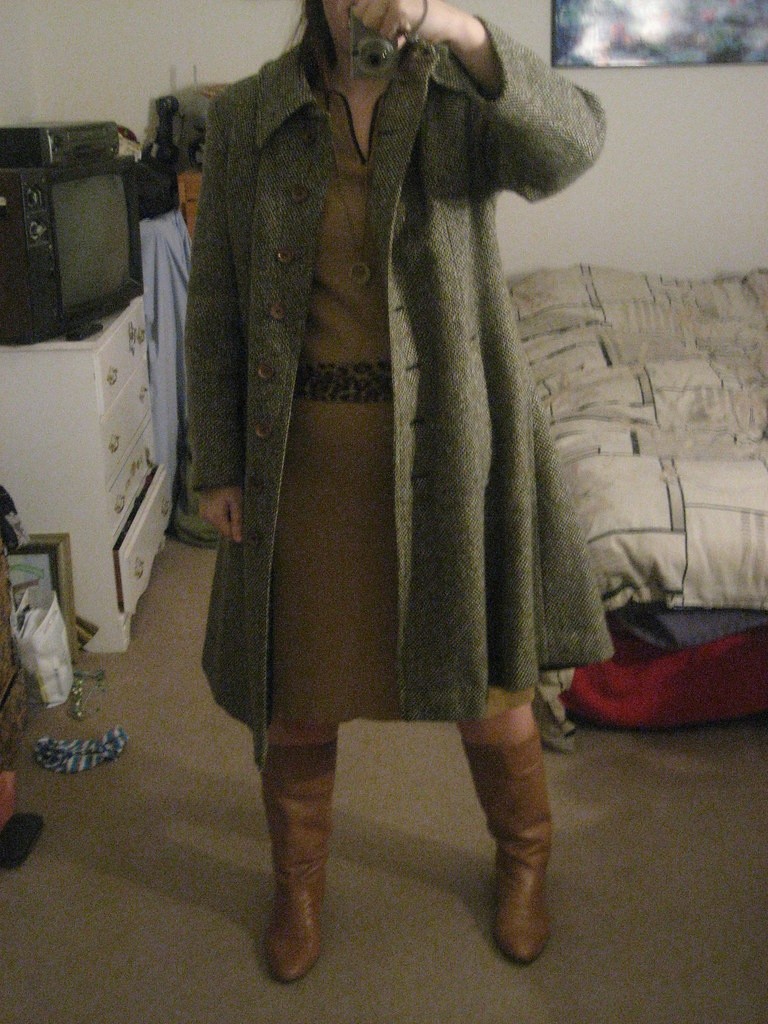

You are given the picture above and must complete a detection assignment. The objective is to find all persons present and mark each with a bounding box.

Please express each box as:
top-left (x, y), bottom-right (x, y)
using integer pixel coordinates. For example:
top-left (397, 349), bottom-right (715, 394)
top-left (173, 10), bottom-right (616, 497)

top-left (182, 0), bottom-right (608, 983)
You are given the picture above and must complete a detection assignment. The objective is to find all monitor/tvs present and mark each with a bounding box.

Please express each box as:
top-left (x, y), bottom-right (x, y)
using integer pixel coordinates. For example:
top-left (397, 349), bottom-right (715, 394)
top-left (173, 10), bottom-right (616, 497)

top-left (0, 154), bottom-right (145, 345)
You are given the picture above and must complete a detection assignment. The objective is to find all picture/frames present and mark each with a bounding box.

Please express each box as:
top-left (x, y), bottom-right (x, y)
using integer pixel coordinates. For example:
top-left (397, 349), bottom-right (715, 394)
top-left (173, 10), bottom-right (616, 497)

top-left (5, 532), bottom-right (78, 664)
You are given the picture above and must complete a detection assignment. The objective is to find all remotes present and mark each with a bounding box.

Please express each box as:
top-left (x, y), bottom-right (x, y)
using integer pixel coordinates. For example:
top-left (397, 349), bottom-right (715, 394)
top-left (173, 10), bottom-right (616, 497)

top-left (66, 324), bottom-right (103, 341)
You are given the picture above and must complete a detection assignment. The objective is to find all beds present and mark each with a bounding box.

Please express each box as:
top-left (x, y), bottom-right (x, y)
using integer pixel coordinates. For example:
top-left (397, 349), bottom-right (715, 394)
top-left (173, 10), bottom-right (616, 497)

top-left (503, 265), bottom-right (768, 751)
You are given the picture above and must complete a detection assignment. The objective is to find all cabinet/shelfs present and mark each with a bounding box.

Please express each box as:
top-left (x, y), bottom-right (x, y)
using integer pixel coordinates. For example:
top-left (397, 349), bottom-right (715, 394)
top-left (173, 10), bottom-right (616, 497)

top-left (1, 296), bottom-right (177, 655)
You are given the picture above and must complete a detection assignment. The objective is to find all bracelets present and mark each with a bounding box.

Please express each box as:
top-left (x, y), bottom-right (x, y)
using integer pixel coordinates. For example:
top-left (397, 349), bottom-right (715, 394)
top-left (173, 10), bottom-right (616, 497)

top-left (407, 0), bottom-right (429, 42)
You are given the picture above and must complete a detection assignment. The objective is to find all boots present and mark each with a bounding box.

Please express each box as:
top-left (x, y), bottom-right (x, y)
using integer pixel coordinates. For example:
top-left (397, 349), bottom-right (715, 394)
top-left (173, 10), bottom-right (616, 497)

top-left (254, 739), bottom-right (337, 982)
top-left (460, 736), bottom-right (552, 964)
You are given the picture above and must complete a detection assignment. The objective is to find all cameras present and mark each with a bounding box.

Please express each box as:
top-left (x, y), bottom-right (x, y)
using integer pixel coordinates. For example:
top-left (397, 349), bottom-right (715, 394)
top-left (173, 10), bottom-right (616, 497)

top-left (347, 8), bottom-right (399, 80)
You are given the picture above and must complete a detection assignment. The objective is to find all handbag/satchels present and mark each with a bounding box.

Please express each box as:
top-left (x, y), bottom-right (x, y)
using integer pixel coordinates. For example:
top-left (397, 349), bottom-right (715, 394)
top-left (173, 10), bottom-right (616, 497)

top-left (9, 587), bottom-right (73, 708)
